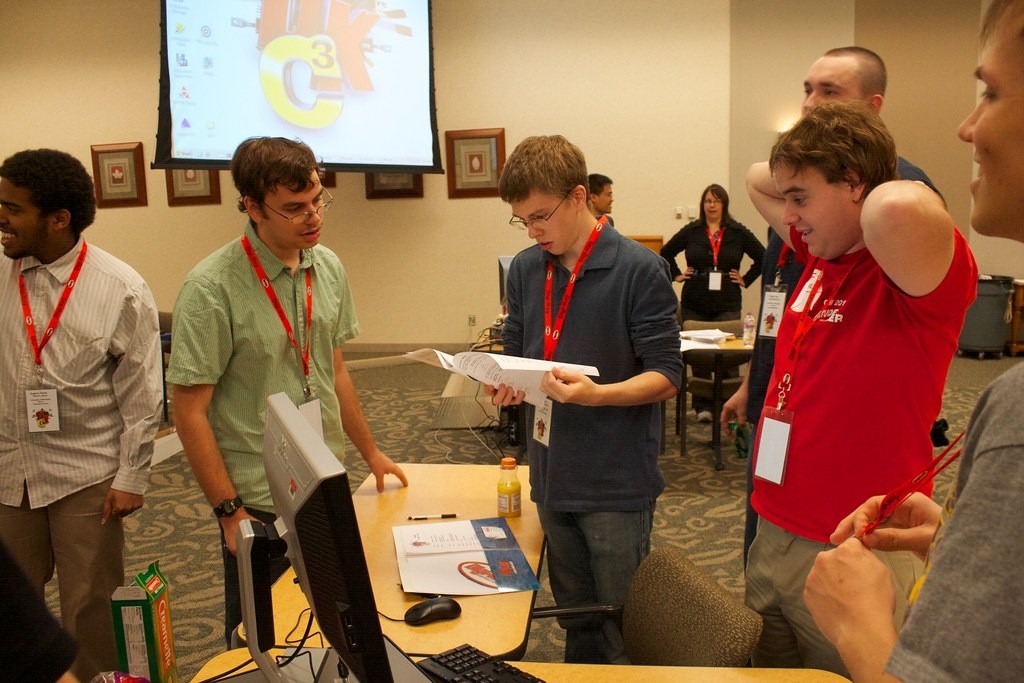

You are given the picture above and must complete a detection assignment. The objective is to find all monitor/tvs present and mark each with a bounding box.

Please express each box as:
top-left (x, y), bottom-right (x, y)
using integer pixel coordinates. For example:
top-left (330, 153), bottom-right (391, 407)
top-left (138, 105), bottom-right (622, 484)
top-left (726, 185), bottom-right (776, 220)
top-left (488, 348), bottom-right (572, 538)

top-left (497, 256), bottom-right (516, 305)
top-left (235, 392), bottom-right (393, 683)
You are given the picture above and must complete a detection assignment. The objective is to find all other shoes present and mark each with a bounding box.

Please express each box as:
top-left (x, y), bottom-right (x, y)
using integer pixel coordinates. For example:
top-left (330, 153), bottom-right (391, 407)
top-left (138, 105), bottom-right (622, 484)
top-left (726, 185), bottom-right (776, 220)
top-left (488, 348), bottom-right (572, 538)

top-left (697, 411), bottom-right (713, 422)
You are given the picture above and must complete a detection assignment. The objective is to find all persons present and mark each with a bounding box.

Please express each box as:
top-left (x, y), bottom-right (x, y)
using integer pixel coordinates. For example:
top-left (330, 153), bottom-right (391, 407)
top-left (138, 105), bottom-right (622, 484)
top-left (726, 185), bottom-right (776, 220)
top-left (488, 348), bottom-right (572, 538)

top-left (0, 148), bottom-right (163, 683)
top-left (660, 184), bottom-right (765, 424)
top-left (745, 99), bottom-right (978, 678)
top-left (1, 543), bottom-right (82, 683)
top-left (719, 44), bottom-right (947, 578)
top-left (587, 173), bottom-right (615, 231)
top-left (485, 135), bottom-right (684, 665)
top-left (802, 1), bottom-right (1024, 683)
top-left (165, 136), bottom-right (406, 650)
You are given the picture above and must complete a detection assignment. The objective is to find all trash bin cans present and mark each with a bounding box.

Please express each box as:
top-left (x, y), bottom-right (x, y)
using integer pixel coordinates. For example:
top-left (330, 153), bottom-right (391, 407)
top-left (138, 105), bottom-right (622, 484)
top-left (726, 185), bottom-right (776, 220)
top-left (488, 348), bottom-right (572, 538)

top-left (957, 274), bottom-right (1014, 359)
top-left (1006, 280), bottom-right (1024, 357)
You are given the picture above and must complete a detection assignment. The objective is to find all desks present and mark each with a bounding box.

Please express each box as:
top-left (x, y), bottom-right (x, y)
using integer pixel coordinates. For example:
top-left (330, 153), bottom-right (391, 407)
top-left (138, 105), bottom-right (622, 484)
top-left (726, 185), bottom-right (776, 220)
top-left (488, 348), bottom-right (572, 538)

top-left (188, 648), bottom-right (855, 683)
top-left (238, 463), bottom-right (546, 661)
top-left (678, 331), bottom-right (755, 471)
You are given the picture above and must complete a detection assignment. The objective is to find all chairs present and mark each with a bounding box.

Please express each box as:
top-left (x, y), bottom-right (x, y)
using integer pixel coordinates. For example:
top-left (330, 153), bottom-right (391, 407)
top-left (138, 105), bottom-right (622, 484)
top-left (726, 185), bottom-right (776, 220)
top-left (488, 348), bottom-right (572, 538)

top-left (675, 319), bottom-right (749, 436)
top-left (532, 546), bottom-right (763, 664)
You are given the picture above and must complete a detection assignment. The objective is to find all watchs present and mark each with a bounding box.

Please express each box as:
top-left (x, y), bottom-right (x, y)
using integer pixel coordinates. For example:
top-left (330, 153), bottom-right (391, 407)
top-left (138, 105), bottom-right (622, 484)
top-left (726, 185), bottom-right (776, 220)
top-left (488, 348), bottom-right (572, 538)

top-left (213, 495), bottom-right (244, 519)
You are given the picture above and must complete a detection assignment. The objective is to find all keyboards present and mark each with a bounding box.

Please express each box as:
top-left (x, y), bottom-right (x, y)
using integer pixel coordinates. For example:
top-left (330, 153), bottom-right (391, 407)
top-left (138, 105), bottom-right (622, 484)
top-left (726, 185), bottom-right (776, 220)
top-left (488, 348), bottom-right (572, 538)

top-left (417, 643), bottom-right (547, 683)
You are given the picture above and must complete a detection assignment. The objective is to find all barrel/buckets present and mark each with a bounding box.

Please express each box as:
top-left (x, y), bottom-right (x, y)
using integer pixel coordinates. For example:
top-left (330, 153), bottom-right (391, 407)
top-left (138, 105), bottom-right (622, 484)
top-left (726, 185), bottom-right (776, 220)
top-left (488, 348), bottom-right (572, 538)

top-left (955, 274), bottom-right (1014, 359)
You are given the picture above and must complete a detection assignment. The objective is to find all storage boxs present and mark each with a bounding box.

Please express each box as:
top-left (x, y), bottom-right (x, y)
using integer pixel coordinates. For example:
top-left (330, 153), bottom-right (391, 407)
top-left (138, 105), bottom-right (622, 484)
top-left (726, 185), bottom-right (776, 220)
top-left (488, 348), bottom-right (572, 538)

top-left (110, 560), bottom-right (178, 683)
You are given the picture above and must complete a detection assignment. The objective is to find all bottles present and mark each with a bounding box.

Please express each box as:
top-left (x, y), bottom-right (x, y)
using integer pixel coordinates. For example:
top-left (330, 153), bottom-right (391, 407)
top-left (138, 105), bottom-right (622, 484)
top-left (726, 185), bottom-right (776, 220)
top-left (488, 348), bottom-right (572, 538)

top-left (743, 312), bottom-right (756, 345)
top-left (497, 457), bottom-right (522, 518)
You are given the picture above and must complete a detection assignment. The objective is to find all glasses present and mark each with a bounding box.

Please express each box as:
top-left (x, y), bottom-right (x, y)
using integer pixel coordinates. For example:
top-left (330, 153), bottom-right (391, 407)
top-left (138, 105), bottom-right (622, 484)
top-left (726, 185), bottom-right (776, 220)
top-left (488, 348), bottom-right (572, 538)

top-left (509, 190), bottom-right (572, 230)
top-left (262, 185), bottom-right (334, 225)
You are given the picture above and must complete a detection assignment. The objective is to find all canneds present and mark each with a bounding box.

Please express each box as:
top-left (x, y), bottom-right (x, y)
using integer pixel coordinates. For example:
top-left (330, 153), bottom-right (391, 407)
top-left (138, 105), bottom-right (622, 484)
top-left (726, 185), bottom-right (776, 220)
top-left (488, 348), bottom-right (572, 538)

top-left (728, 418), bottom-right (752, 459)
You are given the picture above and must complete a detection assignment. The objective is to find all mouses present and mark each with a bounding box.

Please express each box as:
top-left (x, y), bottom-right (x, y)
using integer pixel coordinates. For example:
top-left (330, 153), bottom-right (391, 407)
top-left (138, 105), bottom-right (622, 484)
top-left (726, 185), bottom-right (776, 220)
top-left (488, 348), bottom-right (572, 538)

top-left (404, 598), bottom-right (461, 625)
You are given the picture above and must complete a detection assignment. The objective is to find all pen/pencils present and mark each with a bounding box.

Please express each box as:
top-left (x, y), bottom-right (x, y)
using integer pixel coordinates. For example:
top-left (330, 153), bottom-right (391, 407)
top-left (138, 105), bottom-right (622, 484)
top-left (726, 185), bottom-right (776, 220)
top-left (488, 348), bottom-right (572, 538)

top-left (407, 513), bottom-right (460, 521)
top-left (396, 583), bottom-right (443, 600)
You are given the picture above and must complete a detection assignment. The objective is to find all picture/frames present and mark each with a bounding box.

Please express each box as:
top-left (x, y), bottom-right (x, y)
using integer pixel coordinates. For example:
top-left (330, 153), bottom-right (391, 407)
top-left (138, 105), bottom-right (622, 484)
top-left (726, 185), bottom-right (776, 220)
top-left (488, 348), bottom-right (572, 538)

top-left (364, 171), bottom-right (424, 200)
top-left (90, 142), bottom-right (148, 210)
top-left (318, 171), bottom-right (336, 188)
top-left (165, 169), bottom-right (221, 207)
top-left (444, 128), bottom-right (506, 199)
